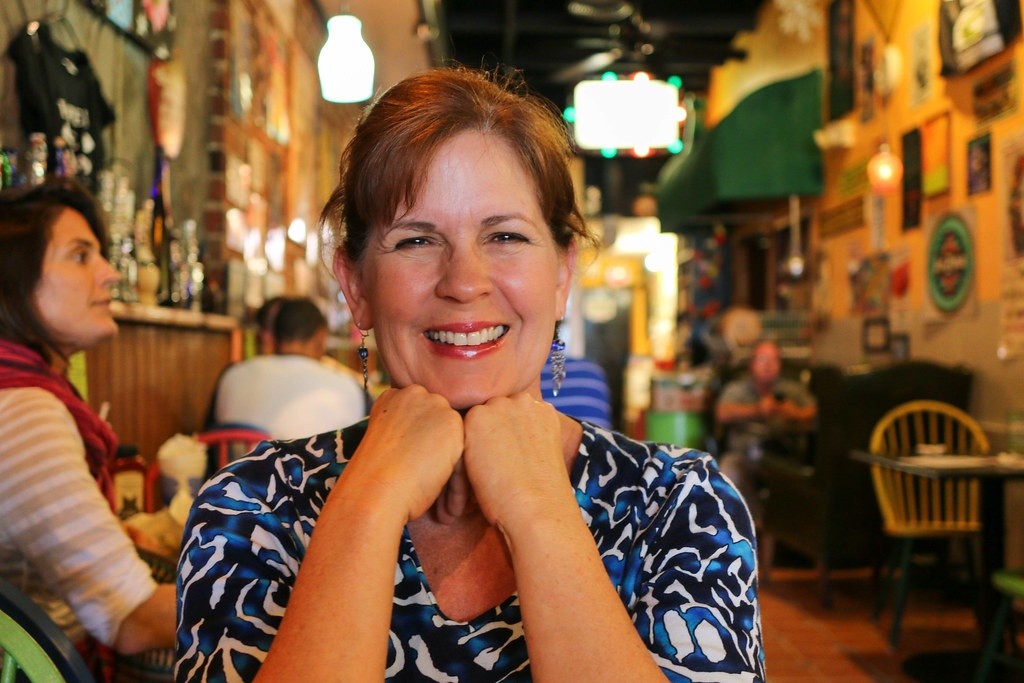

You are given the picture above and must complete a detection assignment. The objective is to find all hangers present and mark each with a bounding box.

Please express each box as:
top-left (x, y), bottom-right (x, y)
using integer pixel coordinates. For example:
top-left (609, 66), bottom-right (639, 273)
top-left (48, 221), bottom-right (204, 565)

top-left (40, 0), bottom-right (81, 50)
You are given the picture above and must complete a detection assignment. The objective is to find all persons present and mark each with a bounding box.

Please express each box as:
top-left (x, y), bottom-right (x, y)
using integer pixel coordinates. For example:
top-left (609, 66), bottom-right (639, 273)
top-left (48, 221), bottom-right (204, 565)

top-left (0, 189), bottom-right (179, 683)
top-left (715, 341), bottom-right (818, 539)
top-left (173, 69), bottom-right (767, 683)
top-left (200, 294), bottom-right (374, 486)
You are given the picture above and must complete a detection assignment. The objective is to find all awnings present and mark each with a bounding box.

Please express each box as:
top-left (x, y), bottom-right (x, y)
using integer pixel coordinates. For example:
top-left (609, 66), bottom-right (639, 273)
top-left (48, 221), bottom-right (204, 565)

top-left (654, 68), bottom-right (823, 236)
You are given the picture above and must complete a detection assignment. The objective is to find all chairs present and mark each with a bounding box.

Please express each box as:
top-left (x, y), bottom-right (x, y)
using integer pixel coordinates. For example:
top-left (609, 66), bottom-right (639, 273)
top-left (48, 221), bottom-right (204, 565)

top-left (972, 569), bottom-right (1024, 683)
top-left (763, 357), bottom-right (975, 610)
top-left (869, 400), bottom-right (992, 650)
top-left (145, 430), bottom-right (271, 514)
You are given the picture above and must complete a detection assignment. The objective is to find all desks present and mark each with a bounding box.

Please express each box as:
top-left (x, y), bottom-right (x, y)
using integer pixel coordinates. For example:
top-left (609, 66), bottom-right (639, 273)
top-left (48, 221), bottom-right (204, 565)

top-left (65, 301), bottom-right (245, 464)
top-left (847, 448), bottom-right (1024, 683)
